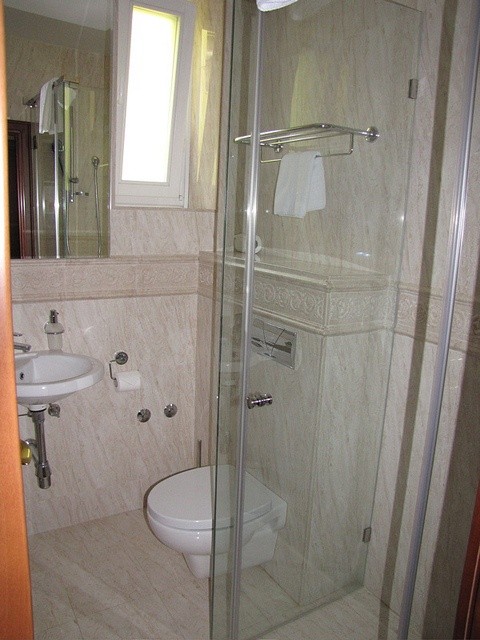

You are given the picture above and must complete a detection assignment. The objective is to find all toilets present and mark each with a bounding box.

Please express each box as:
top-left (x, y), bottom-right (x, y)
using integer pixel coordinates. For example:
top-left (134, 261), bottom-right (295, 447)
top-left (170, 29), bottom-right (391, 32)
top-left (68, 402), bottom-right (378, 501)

top-left (142, 462), bottom-right (288, 580)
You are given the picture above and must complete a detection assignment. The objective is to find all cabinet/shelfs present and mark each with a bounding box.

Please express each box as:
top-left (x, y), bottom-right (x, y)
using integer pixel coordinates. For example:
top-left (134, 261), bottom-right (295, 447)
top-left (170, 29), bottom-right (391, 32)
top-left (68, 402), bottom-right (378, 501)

top-left (214, 301), bottom-right (327, 514)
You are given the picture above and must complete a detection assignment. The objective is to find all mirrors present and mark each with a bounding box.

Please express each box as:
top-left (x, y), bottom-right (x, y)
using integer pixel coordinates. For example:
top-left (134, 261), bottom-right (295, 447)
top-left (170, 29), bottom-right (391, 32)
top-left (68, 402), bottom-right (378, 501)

top-left (5, 0), bottom-right (116, 263)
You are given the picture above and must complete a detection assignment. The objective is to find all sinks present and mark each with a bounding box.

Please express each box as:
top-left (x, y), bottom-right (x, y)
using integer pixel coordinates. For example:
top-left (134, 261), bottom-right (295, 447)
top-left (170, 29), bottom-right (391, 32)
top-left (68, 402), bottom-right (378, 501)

top-left (13, 346), bottom-right (106, 415)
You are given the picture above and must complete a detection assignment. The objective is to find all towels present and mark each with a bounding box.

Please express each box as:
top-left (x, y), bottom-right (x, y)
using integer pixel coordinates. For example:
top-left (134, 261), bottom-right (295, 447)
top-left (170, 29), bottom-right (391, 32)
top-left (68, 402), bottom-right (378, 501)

top-left (272, 149), bottom-right (325, 218)
top-left (37, 77), bottom-right (76, 132)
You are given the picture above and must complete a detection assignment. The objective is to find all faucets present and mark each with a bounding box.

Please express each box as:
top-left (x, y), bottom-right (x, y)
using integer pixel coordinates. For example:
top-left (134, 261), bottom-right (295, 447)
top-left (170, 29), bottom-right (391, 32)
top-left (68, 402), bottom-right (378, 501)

top-left (11, 330), bottom-right (30, 353)
